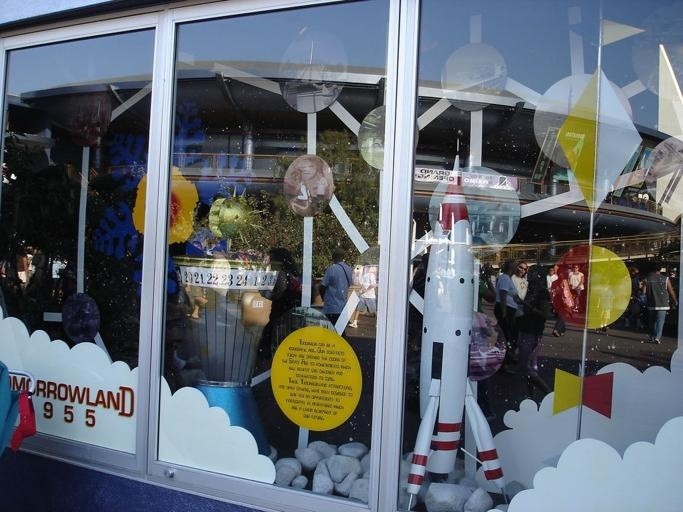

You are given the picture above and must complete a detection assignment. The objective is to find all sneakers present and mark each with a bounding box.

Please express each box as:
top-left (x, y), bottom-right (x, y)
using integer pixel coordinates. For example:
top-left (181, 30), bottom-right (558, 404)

top-left (654, 338), bottom-right (660, 344)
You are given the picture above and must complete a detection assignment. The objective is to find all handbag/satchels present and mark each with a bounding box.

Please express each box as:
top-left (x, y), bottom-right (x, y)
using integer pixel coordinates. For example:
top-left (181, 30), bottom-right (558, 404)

top-left (561, 286), bottom-right (574, 308)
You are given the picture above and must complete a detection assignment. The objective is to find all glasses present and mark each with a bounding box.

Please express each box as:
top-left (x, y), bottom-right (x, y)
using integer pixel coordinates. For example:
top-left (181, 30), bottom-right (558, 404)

top-left (518, 266), bottom-right (526, 270)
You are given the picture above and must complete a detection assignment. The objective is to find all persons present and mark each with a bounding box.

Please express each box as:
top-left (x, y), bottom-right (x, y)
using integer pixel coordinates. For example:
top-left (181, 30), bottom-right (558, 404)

top-left (183, 256), bottom-right (208, 319)
top-left (347, 263), bottom-right (377, 329)
top-left (317, 248), bottom-right (352, 337)
top-left (476, 257), bottom-right (677, 375)
top-left (284, 152), bottom-right (333, 216)
top-left (203, 246), bottom-right (238, 338)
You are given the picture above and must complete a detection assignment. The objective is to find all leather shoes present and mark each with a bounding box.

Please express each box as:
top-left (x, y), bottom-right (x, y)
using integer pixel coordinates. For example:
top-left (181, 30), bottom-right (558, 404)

top-left (553, 330), bottom-right (560, 337)
